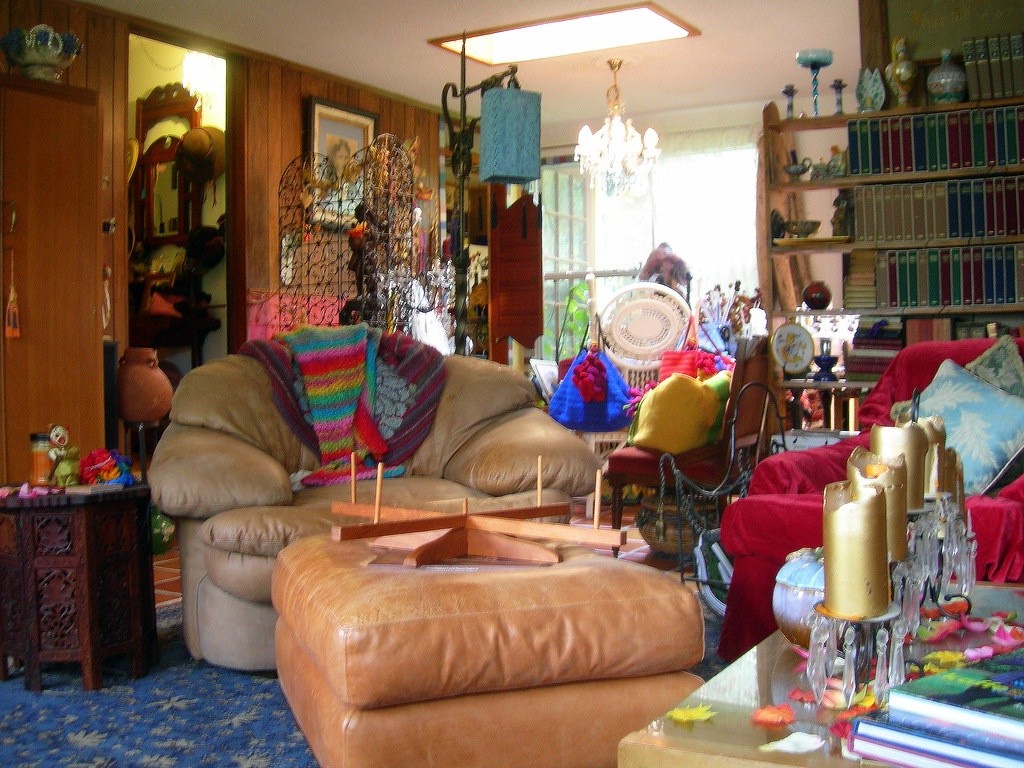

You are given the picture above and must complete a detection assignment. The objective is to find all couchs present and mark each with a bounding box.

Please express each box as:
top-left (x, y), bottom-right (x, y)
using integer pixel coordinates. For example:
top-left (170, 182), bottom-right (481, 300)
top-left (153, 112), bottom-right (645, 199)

top-left (148, 353), bottom-right (600, 675)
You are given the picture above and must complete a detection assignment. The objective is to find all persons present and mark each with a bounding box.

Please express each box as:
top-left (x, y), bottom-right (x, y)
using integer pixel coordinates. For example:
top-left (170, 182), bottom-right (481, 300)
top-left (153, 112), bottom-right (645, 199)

top-left (48, 425), bottom-right (79, 486)
top-left (640, 243), bottom-right (686, 294)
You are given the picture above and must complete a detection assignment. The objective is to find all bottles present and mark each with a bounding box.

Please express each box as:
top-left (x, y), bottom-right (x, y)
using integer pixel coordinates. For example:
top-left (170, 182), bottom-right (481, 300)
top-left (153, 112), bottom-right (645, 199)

top-left (31, 434), bottom-right (55, 485)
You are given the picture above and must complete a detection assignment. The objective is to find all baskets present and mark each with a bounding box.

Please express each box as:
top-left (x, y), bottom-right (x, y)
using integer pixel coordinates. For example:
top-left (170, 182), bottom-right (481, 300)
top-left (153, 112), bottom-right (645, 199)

top-left (634, 495), bottom-right (725, 558)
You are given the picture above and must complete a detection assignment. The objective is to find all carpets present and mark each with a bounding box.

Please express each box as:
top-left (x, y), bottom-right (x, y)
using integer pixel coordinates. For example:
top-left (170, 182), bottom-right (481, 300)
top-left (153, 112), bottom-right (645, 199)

top-left (0, 602), bottom-right (321, 767)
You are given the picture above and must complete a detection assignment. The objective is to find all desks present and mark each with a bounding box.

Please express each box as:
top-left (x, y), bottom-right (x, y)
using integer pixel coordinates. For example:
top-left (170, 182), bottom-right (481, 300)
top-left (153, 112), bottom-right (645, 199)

top-left (3, 481), bottom-right (154, 692)
top-left (617, 617), bottom-right (978, 767)
top-left (772, 382), bottom-right (880, 433)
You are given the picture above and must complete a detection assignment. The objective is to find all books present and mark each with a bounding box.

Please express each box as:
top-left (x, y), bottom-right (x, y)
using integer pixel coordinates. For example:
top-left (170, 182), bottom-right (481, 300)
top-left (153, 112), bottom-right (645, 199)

top-left (845, 31), bottom-right (1024, 308)
top-left (846, 317), bottom-right (1024, 380)
top-left (65, 483), bottom-right (123, 495)
top-left (830, 395), bottom-right (860, 430)
top-left (849, 647), bottom-right (1023, 768)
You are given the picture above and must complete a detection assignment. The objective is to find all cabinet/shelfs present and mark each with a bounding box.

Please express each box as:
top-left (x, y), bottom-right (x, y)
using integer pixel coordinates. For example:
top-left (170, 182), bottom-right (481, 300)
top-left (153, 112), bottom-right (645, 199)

top-left (757, 100), bottom-right (1024, 376)
top-left (0, 72), bottom-right (106, 488)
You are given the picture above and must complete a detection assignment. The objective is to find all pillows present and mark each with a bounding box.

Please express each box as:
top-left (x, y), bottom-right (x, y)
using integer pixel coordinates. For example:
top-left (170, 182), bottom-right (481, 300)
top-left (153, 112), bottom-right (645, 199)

top-left (889, 337), bottom-right (1024, 486)
top-left (901, 357), bottom-right (1024, 496)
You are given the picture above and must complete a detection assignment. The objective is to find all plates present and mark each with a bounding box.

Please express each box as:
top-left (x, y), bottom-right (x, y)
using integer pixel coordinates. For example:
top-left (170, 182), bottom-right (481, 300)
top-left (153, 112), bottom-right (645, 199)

top-left (771, 322), bottom-right (816, 374)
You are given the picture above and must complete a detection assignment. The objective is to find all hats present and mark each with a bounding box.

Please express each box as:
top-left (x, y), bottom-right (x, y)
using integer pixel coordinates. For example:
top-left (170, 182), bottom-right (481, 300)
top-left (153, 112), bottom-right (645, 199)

top-left (175, 124), bottom-right (226, 207)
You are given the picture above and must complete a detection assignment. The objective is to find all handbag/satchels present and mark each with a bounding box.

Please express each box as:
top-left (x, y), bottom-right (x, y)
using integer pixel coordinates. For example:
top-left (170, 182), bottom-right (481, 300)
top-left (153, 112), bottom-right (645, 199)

top-left (546, 313), bottom-right (634, 432)
top-left (626, 370), bottom-right (732, 455)
top-left (478, 74), bottom-right (544, 185)
top-left (656, 313), bottom-right (735, 382)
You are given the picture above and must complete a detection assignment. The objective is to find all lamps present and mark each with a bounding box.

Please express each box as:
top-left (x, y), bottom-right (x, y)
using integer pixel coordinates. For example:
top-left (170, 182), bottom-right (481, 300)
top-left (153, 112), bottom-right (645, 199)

top-left (821, 481), bottom-right (888, 620)
top-left (895, 389), bottom-right (943, 494)
top-left (440, 61), bottom-right (542, 358)
top-left (845, 444), bottom-right (908, 559)
top-left (870, 415), bottom-right (929, 510)
top-left (573, 57), bottom-right (661, 195)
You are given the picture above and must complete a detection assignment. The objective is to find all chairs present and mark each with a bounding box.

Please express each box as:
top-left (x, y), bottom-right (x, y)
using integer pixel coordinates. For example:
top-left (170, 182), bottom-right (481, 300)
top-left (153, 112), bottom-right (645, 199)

top-left (604, 337), bottom-right (768, 560)
top-left (715, 335), bottom-right (1024, 662)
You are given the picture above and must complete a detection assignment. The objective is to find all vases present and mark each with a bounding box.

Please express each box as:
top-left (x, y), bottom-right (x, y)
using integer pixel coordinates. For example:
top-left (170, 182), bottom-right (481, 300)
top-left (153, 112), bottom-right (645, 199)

top-left (883, 38), bottom-right (918, 107)
top-left (927, 48), bottom-right (968, 105)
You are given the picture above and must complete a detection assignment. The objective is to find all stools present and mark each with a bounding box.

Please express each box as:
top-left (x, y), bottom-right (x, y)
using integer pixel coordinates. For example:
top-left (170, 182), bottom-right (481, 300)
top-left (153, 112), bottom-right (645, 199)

top-left (269, 530), bottom-right (707, 768)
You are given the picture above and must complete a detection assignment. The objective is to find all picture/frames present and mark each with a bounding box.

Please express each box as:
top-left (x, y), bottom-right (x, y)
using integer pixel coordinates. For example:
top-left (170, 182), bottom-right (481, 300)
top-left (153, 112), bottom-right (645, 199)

top-left (306, 94), bottom-right (378, 232)
